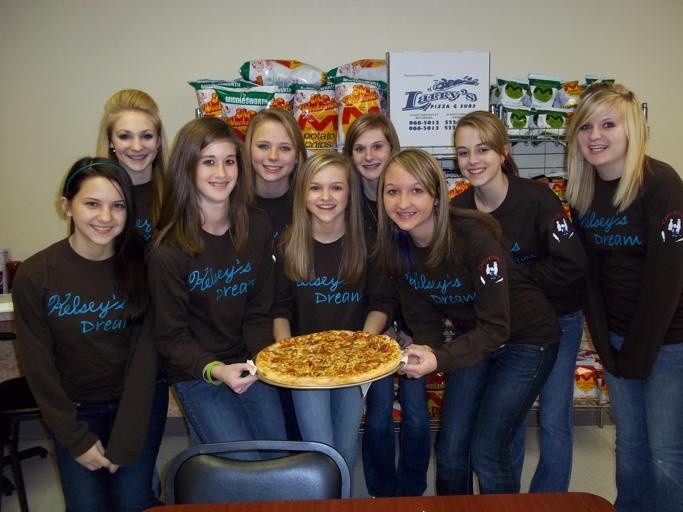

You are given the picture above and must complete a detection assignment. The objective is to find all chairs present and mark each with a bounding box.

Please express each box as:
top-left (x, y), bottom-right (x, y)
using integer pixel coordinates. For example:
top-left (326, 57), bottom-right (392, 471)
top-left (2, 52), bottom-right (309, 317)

top-left (162, 439), bottom-right (351, 506)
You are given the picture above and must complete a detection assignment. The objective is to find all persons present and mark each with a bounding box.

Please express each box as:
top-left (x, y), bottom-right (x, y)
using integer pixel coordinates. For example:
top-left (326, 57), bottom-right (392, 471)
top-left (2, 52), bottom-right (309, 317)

top-left (269, 152), bottom-right (395, 503)
top-left (446, 111), bottom-right (586, 493)
top-left (95, 86), bottom-right (173, 257)
top-left (340, 112), bottom-right (432, 497)
top-left (562, 81), bottom-right (681, 511)
top-left (243, 104), bottom-right (308, 246)
top-left (10, 155), bottom-right (167, 511)
top-left (356, 148), bottom-right (561, 494)
top-left (146, 114), bottom-right (290, 461)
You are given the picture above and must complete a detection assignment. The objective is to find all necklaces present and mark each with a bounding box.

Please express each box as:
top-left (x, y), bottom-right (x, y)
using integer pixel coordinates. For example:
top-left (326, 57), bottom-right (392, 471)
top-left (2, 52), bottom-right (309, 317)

top-left (361, 194), bottom-right (379, 227)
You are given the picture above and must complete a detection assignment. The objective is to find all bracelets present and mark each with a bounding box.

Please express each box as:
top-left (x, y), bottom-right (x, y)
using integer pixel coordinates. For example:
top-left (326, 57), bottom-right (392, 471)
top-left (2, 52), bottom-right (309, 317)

top-left (204, 360), bottom-right (227, 389)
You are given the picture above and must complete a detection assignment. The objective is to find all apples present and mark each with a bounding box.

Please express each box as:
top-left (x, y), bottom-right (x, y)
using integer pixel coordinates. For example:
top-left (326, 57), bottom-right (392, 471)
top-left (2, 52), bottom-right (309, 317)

top-left (534, 86), bottom-right (553, 103)
top-left (546, 114), bottom-right (563, 128)
top-left (511, 112), bottom-right (527, 128)
top-left (505, 84), bottom-right (523, 99)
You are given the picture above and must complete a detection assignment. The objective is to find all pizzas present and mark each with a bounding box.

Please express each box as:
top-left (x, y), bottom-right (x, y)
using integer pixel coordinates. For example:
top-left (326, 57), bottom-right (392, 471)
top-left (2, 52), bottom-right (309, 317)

top-left (256, 330), bottom-right (402, 386)
